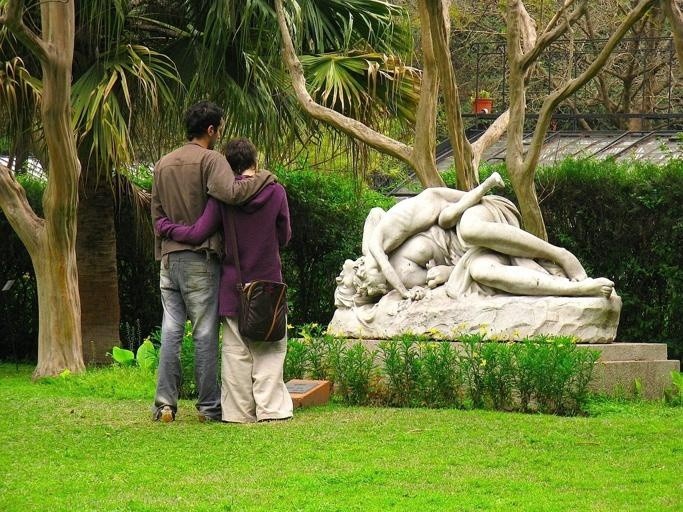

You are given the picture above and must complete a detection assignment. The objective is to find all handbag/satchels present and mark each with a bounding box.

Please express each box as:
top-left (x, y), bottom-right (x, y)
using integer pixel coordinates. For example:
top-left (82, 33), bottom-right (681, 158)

top-left (237, 278), bottom-right (290, 343)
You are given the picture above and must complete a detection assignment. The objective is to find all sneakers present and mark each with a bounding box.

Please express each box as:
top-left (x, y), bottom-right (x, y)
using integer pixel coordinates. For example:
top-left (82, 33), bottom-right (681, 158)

top-left (155, 404), bottom-right (176, 422)
top-left (196, 411), bottom-right (229, 423)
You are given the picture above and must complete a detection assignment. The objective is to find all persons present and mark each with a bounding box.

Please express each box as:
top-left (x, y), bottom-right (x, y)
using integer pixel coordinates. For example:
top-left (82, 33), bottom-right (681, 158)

top-left (154, 134), bottom-right (296, 425)
top-left (347, 169), bottom-right (507, 301)
top-left (147, 99), bottom-right (281, 424)
top-left (330, 193), bottom-right (616, 313)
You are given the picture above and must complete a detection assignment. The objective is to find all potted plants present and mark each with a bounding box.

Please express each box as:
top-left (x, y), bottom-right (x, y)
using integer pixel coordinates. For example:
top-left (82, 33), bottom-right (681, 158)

top-left (470, 90), bottom-right (494, 115)
